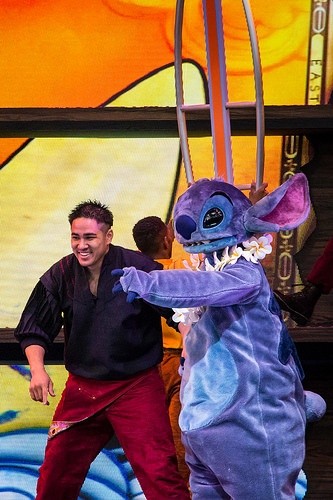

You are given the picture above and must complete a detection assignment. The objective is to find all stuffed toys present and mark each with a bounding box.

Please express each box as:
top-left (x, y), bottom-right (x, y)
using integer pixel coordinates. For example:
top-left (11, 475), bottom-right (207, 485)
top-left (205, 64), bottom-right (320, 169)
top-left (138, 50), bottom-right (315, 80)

top-left (111, 173), bottom-right (326, 500)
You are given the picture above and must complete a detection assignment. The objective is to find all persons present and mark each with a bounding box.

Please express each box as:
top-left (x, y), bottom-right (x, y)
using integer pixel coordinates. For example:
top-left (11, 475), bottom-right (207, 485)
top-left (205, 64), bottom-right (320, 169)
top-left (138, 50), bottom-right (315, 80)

top-left (271, 238), bottom-right (333, 327)
top-left (132, 181), bottom-right (269, 500)
top-left (15, 200), bottom-right (197, 500)
